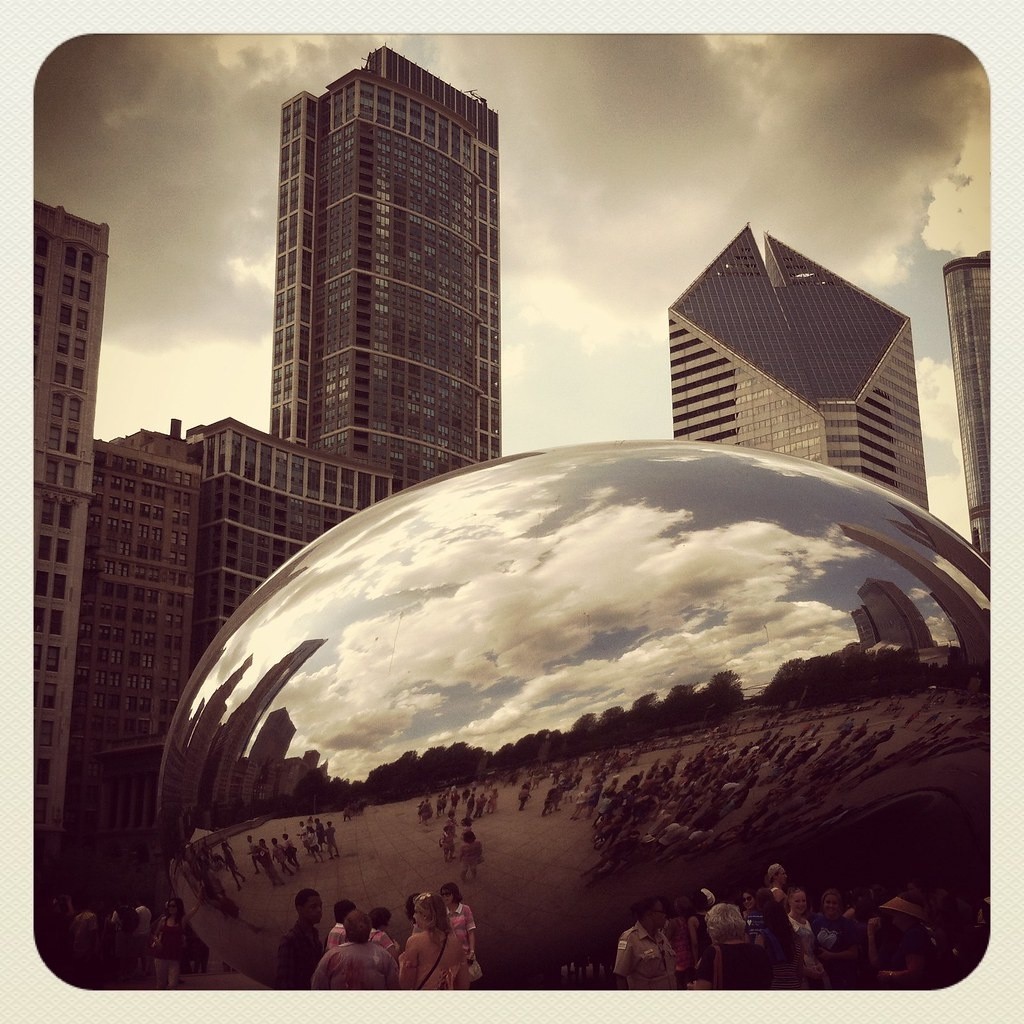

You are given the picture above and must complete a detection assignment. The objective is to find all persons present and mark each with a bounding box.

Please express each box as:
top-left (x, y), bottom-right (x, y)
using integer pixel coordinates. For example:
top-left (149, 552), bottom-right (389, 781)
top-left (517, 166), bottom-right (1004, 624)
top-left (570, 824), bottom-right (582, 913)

top-left (169, 680), bottom-right (990, 991)
top-left (42, 896), bottom-right (209, 990)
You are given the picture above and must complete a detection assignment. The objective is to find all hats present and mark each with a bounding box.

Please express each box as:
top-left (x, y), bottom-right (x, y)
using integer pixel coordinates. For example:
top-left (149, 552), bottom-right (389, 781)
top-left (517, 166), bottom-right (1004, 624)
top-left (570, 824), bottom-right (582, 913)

top-left (768, 863), bottom-right (783, 880)
top-left (879, 897), bottom-right (924, 919)
top-left (701, 888), bottom-right (715, 906)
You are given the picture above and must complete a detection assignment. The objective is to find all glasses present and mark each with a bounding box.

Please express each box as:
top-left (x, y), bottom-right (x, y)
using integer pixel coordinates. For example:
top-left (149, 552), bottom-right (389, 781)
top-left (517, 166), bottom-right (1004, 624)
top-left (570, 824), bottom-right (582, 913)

top-left (440, 892), bottom-right (451, 898)
top-left (742, 896), bottom-right (752, 903)
top-left (653, 909), bottom-right (666, 914)
top-left (167, 904), bottom-right (176, 908)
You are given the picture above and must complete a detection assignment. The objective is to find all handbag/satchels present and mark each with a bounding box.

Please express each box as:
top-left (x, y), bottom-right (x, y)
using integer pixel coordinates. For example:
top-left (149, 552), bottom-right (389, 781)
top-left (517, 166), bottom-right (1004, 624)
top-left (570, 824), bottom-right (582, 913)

top-left (467, 954), bottom-right (482, 982)
